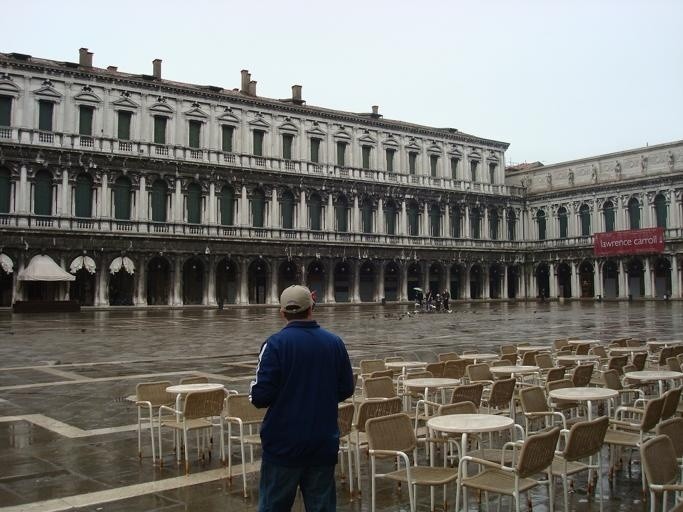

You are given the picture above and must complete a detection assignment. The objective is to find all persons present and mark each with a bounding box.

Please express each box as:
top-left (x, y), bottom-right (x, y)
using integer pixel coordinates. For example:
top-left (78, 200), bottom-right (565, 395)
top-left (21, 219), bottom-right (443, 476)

top-left (247, 284), bottom-right (355, 512)
top-left (539, 288), bottom-right (547, 306)
top-left (627, 287), bottom-right (633, 304)
top-left (414, 287), bottom-right (451, 313)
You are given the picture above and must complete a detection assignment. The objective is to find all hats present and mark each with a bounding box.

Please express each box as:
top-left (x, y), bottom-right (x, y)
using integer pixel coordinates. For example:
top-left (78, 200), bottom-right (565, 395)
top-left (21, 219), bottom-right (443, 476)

top-left (280, 284), bottom-right (313, 313)
